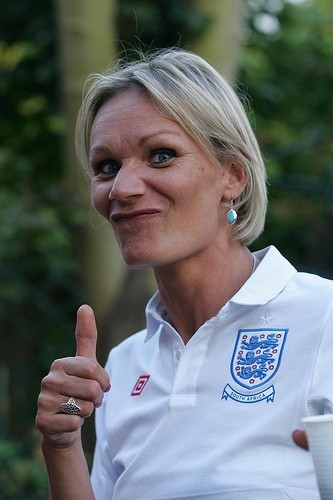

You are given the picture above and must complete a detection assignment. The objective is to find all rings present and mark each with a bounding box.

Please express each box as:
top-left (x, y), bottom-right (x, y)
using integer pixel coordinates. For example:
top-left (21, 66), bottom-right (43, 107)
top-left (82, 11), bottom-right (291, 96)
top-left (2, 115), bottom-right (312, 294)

top-left (59, 396), bottom-right (82, 415)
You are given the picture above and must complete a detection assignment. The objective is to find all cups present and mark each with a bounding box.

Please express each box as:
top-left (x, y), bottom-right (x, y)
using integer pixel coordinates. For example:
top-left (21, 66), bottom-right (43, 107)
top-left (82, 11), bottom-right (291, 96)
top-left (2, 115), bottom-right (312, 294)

top-left (302, 414), bottom-right (333, 500)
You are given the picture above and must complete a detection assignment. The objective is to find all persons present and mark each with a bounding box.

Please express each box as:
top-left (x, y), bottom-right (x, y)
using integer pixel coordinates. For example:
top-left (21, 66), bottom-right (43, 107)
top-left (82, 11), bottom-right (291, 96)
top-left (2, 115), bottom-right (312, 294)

top-left (34, 47), bottom-right (333, 500)
